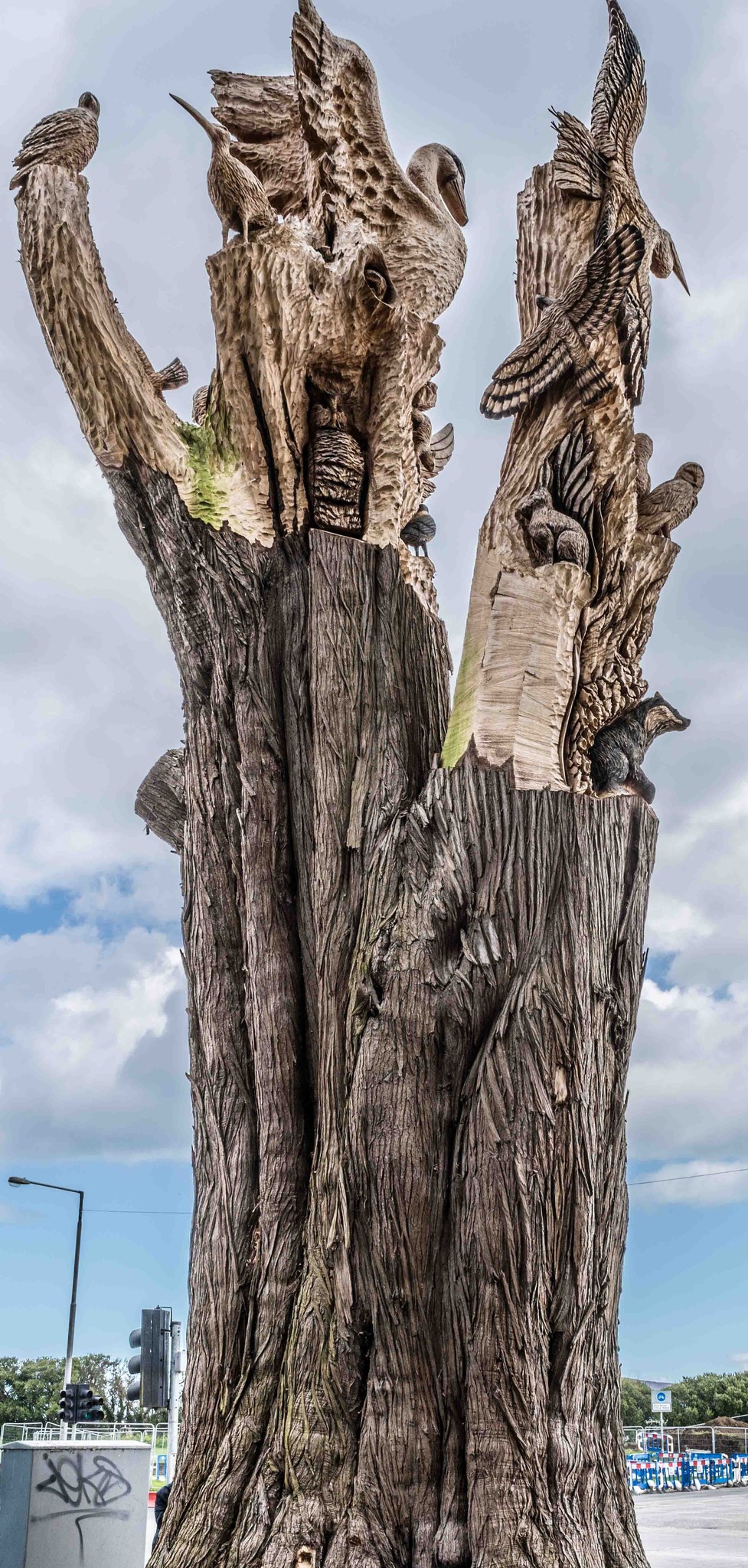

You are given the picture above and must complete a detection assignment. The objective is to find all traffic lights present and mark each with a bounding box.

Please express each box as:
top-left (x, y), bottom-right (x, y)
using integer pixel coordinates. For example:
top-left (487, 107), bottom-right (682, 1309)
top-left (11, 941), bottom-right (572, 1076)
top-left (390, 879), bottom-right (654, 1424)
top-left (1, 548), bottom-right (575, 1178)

top-left (89, 1395), bottom-right (104, 1423)
top-left (76, 1384), bottom-right (93, 1422)
top-left (127, 1309), bottom-right (169, 1409)
top-left (60, 1384), bottom-right (77, 1423)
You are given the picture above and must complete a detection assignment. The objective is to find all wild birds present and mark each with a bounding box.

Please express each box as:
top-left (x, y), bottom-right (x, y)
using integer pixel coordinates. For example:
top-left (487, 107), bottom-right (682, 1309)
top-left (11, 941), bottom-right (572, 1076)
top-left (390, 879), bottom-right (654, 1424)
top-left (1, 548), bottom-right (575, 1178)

top-left (208, 0), bottom-right (469, 320)
top-left (168, 91), bottom-right (279, 247)
top-left (547, 0), bottom-right (693, 296)
top-left (633, 434), bottom-right (707, 536)
top-left (9, 91), bottom-right (100, 191)
top-left (480, 227), bottom-right (647, 418)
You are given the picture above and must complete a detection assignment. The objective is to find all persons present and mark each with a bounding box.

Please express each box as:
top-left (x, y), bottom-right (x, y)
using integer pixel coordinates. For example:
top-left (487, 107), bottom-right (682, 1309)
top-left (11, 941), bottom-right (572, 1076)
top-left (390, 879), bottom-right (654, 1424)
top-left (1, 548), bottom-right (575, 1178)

top-left (151, 1475), bottom-right (175, 1553)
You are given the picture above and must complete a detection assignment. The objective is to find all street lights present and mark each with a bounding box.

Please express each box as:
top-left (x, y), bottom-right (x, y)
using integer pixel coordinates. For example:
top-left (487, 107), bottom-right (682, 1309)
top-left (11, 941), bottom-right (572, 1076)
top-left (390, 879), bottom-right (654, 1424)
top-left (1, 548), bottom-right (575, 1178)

top-left (8, 1176), bottom-right (84, 1441)
